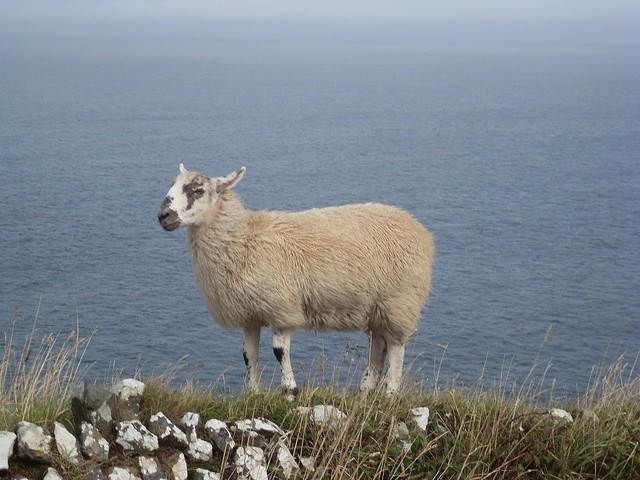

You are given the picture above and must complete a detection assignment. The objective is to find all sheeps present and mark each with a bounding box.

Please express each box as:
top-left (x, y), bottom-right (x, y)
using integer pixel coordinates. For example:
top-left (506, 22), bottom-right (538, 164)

top-left (157, 161), bottom-right (433, 406)
